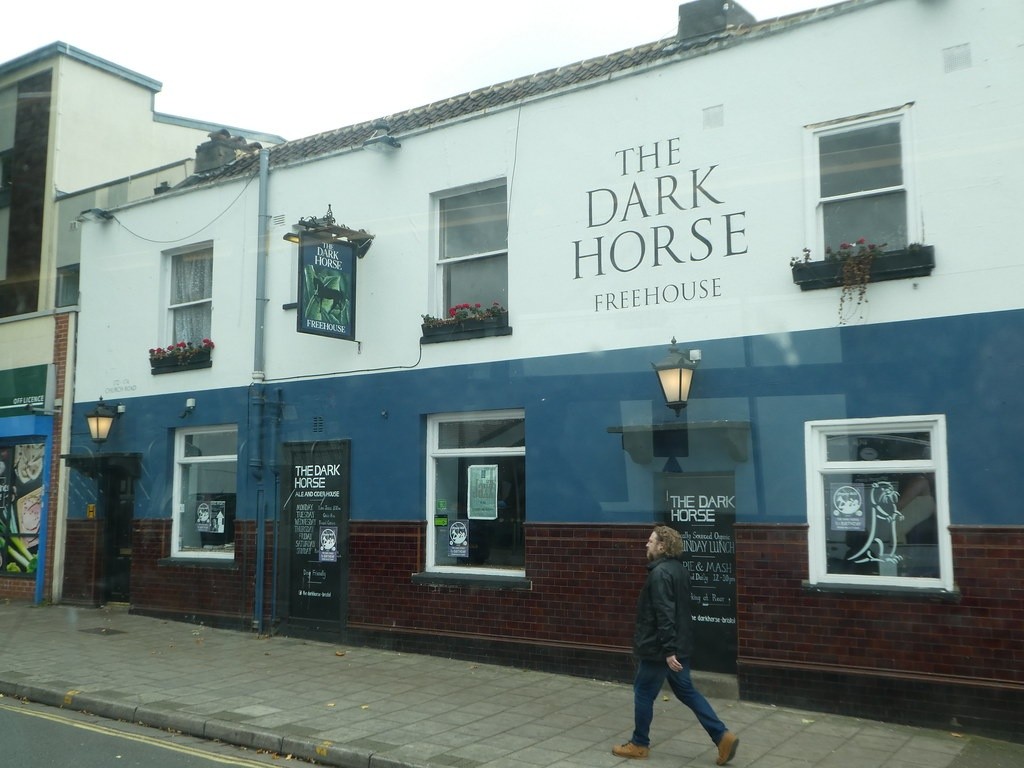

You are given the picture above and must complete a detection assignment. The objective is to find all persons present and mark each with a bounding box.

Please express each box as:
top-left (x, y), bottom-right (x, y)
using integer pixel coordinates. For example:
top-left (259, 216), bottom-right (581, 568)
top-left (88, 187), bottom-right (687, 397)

top-left (612, 526), bottom-right (739, 766)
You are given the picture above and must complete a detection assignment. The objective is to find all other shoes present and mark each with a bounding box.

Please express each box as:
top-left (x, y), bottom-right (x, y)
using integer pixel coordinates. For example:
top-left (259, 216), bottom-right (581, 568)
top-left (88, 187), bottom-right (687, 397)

top-left (612, 740), bottom-right (649, 760)
top-left (717, 732), bottom-right (740, 766)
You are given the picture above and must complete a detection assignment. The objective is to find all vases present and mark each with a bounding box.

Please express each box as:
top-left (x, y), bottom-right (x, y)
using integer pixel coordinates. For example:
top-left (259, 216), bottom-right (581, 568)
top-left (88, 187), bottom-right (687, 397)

top-left (150, 351), bottom-right (210, 367)
top-left (791, 245), bottom-right (934, 291)
top-left (422, 314), bottom-right (505, 337)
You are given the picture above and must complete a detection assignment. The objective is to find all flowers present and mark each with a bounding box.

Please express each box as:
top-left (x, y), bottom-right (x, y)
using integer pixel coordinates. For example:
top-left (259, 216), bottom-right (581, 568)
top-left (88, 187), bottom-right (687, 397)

top-left (826, 238), bottom-right (883, 260)
top-left (420, 302), bottom-right (505, 328)
top-left (149, 338), bottom-right (215, 365)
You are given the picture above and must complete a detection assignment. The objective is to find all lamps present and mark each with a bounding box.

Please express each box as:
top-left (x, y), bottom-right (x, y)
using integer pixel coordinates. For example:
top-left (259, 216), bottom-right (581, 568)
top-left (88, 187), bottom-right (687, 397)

top-left (80, 208), bottom-right (113, 222)
top-left (84, 395), bottom-right (120, 452)
top-left (650, 337), bottom-right (697, 417)
top-left (363, 136), bottom-right (401, 152)
top-left (283, 232), bottom-right (299, 244)
